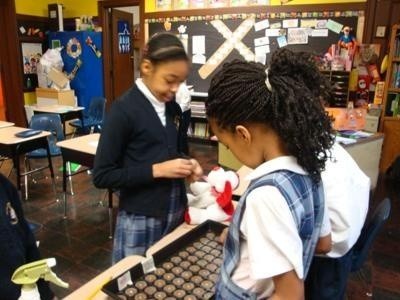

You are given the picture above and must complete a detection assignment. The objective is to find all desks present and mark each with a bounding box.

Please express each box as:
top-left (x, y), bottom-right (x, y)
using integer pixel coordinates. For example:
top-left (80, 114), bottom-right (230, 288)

top-left (33, 105), bottom-right (85, 141)
top-left (0, 120), bottom-right (54, 191)
top-left (55, 133), bottom-right (121, 209)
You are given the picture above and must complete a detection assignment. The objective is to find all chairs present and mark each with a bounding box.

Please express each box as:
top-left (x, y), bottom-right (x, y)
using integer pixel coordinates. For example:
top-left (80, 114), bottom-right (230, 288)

top-left (23, 113), bottom-right (75, 201)
top-left (68, 96), bottom-right (107, 140)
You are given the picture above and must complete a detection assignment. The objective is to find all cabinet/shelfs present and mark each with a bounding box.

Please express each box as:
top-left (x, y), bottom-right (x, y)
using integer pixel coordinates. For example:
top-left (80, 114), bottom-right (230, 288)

top-left (378, 24), bottom-right (400, 174)
top-left (183, 96), bottom-right (221, 146)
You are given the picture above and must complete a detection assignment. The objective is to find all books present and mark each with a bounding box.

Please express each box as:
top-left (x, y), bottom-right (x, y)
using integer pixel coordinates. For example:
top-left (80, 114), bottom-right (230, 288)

top-left (48, 4), bottom-right (64, 32)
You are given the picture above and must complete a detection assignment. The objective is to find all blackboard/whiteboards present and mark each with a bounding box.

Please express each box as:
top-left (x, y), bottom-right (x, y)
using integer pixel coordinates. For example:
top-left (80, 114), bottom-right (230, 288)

top-left (144, 10), bottom-right (365, 97)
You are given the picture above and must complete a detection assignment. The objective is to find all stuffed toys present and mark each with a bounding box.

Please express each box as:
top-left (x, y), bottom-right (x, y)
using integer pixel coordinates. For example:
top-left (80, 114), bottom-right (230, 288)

top-left (338, 25), bottom-right (357, 49)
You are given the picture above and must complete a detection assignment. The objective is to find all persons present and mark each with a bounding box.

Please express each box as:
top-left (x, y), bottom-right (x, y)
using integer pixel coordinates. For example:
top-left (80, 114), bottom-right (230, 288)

top-left (204, 47), bottom-right (337, 300)
top-left (174, 80), bottom-right (192, 158)
top-left (304, 137), bottom-right (371, 299)
top-left (92, 33), bottom-right (204, 265)
top-left (0, 172), bottom-right (58, 300)
